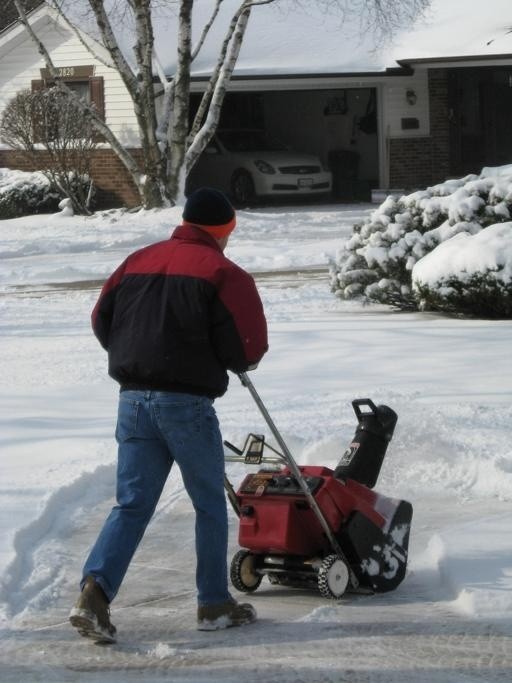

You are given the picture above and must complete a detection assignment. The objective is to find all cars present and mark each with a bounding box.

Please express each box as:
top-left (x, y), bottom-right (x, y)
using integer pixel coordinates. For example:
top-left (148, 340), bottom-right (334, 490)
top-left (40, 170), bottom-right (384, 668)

top-left (186, 125), bottom-right (336, 206)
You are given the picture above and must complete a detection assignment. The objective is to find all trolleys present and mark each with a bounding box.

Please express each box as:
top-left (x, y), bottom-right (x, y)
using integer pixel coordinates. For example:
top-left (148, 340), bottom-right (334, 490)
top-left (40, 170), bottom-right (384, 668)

top-left (221, 366), bottom-right (414, 602)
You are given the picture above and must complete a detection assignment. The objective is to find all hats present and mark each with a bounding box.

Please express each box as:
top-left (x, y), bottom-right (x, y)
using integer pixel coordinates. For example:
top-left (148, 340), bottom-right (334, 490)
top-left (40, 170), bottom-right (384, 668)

top-left (181, 187), bottom-right (237, 239)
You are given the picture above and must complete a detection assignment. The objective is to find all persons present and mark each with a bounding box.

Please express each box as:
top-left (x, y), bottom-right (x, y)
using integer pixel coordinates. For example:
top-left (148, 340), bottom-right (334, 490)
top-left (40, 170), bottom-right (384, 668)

top-left (65, 186), bottom-right (271, 646)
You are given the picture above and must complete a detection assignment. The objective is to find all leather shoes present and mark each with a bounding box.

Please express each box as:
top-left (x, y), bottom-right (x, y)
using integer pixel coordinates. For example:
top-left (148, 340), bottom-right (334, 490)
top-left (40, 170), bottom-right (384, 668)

top-left (196, 602), bottom-right (257, 631)
top-left (68, 576), bottom-right (118, 645)
